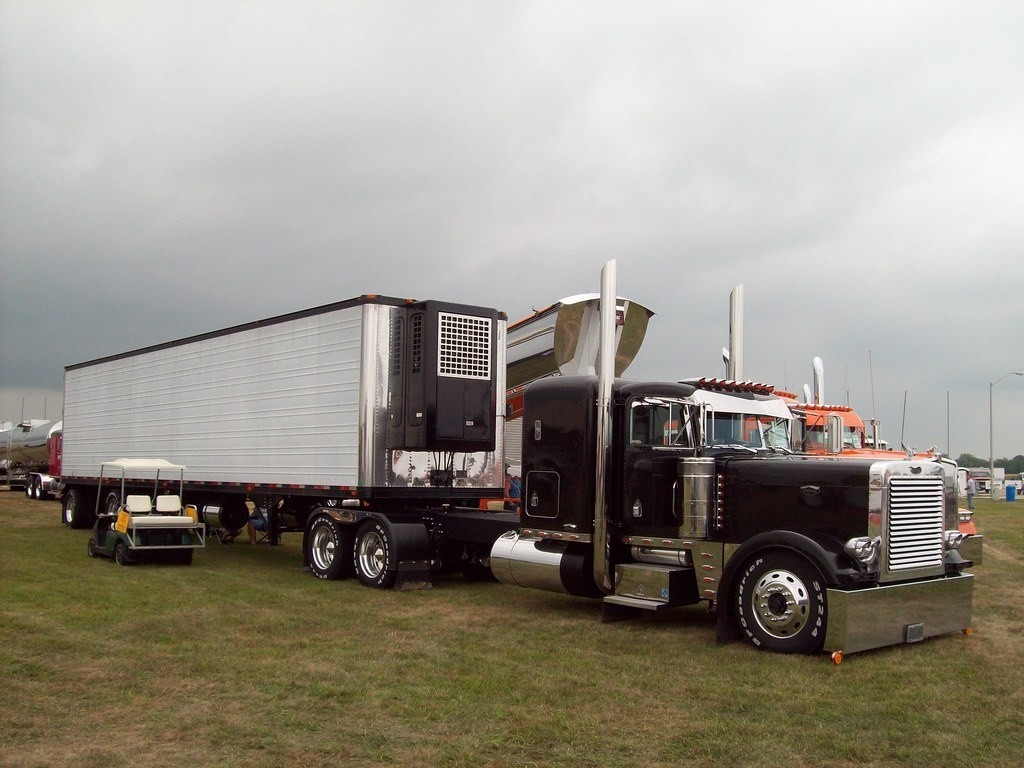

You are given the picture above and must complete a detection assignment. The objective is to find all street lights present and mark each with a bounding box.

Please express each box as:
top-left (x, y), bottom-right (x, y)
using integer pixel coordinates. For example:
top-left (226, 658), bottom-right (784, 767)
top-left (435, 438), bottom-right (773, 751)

top-left (991, 370), bottom-right (1024, 499)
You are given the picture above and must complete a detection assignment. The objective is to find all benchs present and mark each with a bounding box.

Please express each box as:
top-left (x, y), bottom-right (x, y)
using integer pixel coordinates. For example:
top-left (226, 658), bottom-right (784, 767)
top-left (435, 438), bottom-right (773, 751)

top-left (131, 516), bottom-right (194, 527)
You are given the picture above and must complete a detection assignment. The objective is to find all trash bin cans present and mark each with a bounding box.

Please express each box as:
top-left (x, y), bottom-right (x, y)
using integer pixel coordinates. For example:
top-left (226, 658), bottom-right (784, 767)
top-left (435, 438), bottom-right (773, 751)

top-left (1006, 485), bottom-right (1015, 502)
top-left (993, 487), bottom-right (1000, 502)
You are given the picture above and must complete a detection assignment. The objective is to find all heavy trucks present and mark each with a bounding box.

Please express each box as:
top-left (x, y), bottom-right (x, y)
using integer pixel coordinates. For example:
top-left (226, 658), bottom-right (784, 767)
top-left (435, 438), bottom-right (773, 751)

top-left (60, 259), bottom-right (984, 665)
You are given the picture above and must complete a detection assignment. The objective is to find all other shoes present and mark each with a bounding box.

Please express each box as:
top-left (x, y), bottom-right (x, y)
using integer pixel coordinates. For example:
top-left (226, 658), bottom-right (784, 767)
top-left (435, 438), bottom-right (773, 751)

top-left (227, 539), bottom-right (235, 544)
top-left (220, 538), bottom-right (228, 544)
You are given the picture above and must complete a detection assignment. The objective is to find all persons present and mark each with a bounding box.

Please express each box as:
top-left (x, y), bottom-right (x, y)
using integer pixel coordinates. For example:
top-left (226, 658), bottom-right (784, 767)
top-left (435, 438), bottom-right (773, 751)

top-left (964, 475), bottom-right (975, 509)
top-left (218, 505), bottom-right (270, 544)
top-left (504, 474), bottom-right (520, 512)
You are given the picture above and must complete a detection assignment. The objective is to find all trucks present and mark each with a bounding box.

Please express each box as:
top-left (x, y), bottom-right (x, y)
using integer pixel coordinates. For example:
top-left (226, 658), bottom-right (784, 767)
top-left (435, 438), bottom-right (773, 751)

top-left (958, 466), bottom-right (995, 493)
top-left (24, 420), bottom-right (65, 501)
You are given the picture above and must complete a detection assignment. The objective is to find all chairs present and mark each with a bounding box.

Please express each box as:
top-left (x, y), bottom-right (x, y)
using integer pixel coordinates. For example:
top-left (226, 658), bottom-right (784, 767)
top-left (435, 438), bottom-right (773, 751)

top-left (156, 495), bottom-right (182, 512)
top-left (126, 495), bottom-right (153, 512)
